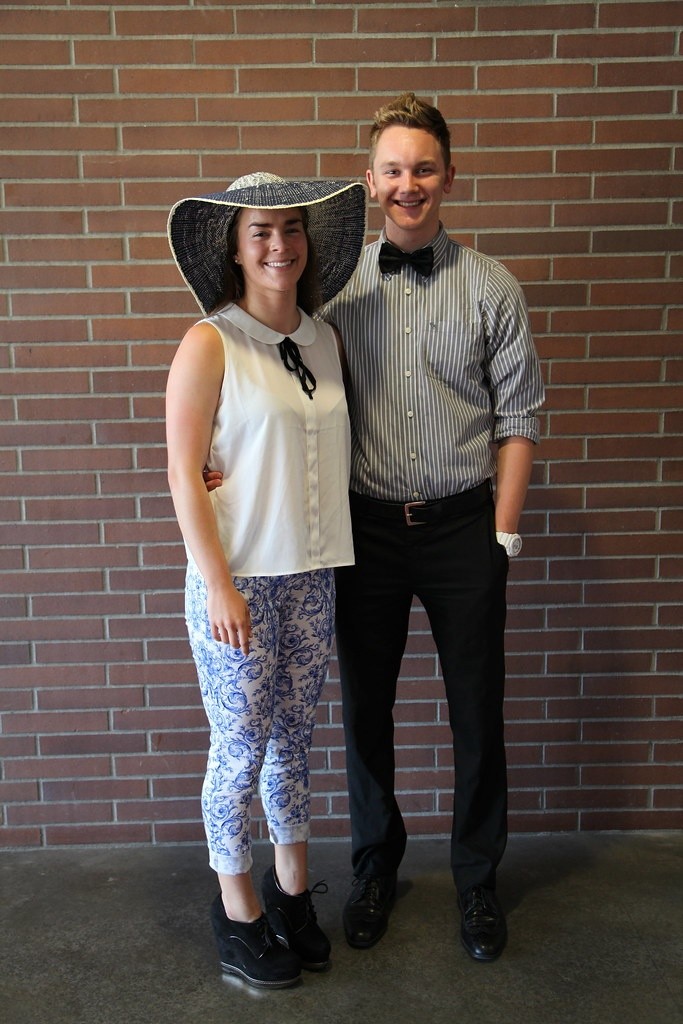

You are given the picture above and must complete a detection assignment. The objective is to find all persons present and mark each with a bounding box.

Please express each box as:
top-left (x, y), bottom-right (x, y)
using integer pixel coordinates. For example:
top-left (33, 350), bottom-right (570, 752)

top-left (165, 172), bottom-right (368, 989)
top-left (202, 93), bottom-right (547, 961)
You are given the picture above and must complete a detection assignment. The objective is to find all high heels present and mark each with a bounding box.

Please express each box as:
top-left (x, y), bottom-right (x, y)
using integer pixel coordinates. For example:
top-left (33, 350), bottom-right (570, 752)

top-left (211, 892), bottom-right (302, 989)
top-left (262, 865), bottom-right (331, 970)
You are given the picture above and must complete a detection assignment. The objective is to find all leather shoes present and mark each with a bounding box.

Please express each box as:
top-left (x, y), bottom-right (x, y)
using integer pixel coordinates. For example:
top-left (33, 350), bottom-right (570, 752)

top-left (458, 890), bottom-right (506, 959)
top-left (343, 878), bottom-right (396, 946)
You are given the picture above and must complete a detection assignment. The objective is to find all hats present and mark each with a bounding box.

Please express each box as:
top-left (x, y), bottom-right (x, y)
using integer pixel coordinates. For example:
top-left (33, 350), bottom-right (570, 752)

top-left (168, 172), bottom-right (369, 318)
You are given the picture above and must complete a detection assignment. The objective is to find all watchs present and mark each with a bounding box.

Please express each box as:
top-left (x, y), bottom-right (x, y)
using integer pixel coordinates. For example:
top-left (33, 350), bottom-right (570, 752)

top-left (494, 533), bottom-right (522, 557)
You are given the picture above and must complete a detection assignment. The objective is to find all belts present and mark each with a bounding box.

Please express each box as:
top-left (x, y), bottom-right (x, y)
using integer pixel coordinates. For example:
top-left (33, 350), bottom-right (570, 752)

top-left (349, 484), bottom-right (491, 526)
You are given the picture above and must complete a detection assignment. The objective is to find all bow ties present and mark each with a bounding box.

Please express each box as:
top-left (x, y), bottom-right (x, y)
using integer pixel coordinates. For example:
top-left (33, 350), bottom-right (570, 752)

top-left (379, 242), bottom-right (434, 277)
